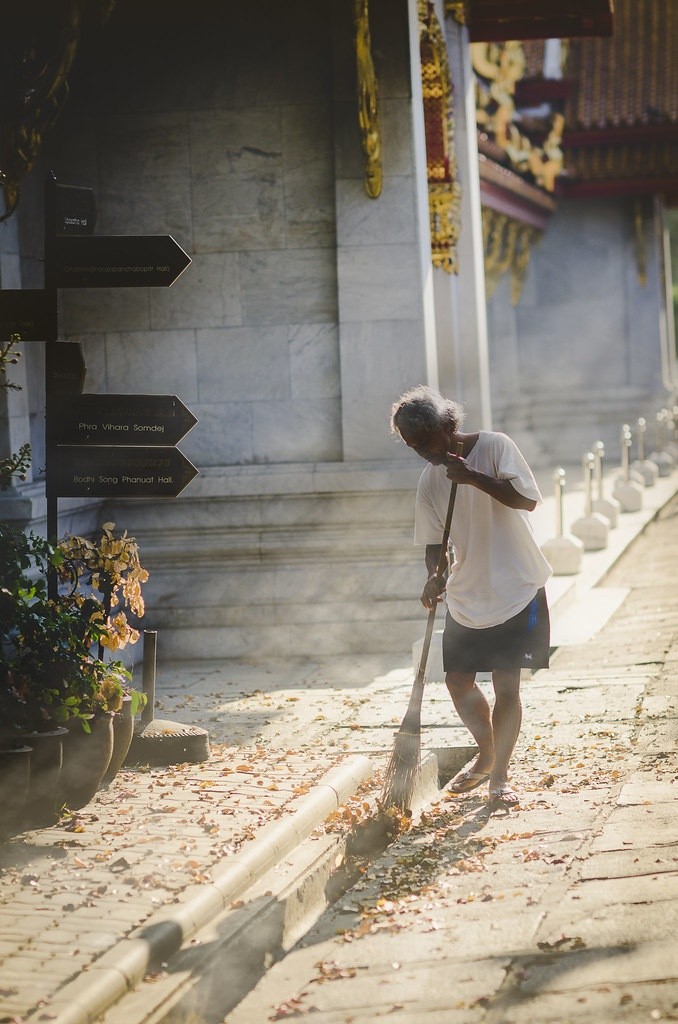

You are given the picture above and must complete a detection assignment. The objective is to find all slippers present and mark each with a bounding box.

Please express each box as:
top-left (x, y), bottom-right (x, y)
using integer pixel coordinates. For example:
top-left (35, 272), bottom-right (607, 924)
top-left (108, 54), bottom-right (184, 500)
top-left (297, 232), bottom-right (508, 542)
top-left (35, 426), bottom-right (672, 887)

top-left (447, 764), bottom-right (510, 793)
top-left (489, 788), bottom-right (519, 807)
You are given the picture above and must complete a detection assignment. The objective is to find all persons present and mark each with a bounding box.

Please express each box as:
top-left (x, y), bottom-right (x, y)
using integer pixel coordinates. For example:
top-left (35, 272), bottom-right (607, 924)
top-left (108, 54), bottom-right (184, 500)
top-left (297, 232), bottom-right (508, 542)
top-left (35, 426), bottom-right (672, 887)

top-left (390, 384), bottom-right (553, 804)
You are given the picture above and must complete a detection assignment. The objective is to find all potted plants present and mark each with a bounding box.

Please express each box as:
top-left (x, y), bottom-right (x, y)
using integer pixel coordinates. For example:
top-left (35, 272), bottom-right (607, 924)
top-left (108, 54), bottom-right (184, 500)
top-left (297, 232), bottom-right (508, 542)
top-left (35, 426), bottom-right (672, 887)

top-left (0, 521), bottom-right (151, 833)
